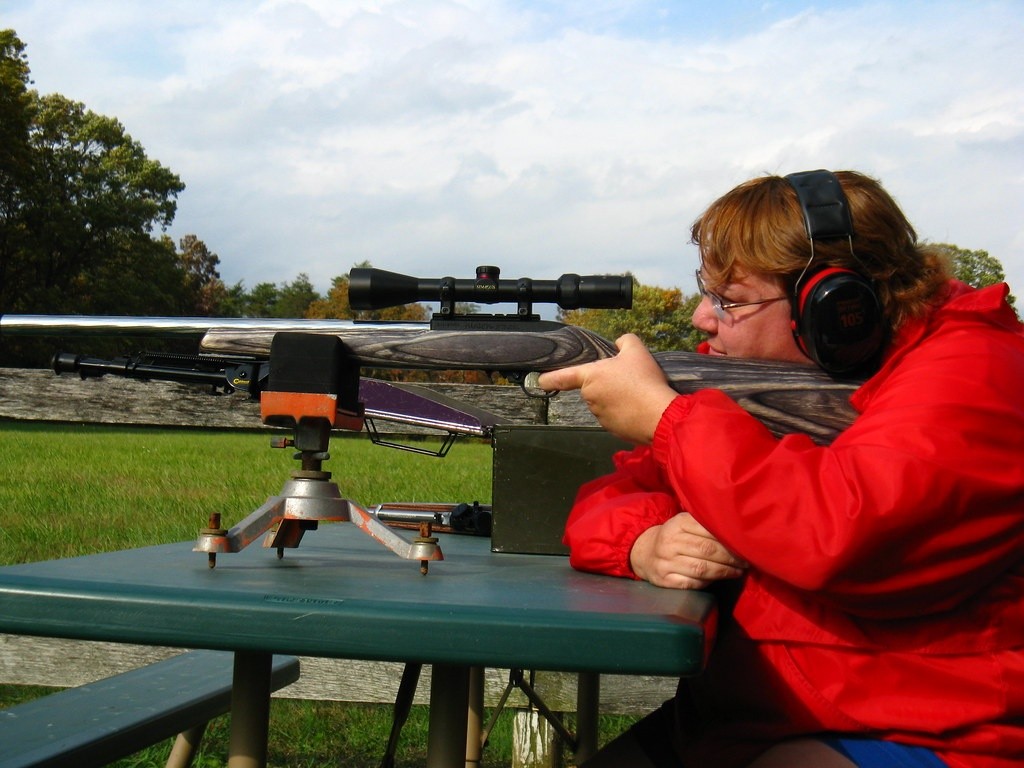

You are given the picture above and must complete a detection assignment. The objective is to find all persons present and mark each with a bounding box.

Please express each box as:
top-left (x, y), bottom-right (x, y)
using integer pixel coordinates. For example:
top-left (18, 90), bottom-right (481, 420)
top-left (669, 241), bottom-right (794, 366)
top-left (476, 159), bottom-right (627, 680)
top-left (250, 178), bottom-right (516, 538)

top-left (538, 171), bottom-right (1024, 768)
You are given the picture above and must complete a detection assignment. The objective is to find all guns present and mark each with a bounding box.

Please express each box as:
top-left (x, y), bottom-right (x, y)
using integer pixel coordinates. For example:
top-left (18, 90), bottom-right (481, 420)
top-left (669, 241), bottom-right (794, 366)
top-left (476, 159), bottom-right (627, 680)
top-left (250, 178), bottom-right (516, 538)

top-left (0, 267), bottom-right (860, 459)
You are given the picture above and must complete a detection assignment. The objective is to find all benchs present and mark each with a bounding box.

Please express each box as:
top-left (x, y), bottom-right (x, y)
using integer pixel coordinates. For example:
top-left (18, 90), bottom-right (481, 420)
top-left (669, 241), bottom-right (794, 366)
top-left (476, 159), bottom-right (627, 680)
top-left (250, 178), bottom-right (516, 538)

top-left (0, 633), bottom-right (682, 768)
top-left (0, 650), bottom-right (300, 768)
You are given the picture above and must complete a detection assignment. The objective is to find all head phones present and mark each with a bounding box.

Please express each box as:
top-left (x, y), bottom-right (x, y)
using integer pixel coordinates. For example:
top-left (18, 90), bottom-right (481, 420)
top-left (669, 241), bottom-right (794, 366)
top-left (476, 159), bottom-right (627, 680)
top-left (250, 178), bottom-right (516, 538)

top-left (784, 169), bottom-right (891, 378)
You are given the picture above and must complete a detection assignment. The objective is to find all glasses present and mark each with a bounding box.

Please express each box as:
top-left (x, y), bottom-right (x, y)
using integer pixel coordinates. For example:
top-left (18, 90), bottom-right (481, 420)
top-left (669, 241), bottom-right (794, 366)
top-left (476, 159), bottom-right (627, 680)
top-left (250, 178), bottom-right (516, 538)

top-left (694, 267), bottom-right (790, 318)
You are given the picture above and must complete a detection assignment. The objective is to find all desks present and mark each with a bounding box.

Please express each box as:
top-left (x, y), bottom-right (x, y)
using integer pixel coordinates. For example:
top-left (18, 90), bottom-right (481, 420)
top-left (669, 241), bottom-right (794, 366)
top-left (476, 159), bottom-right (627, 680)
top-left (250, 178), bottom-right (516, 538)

top-left (0, 522), bottom-right (720, 768)
